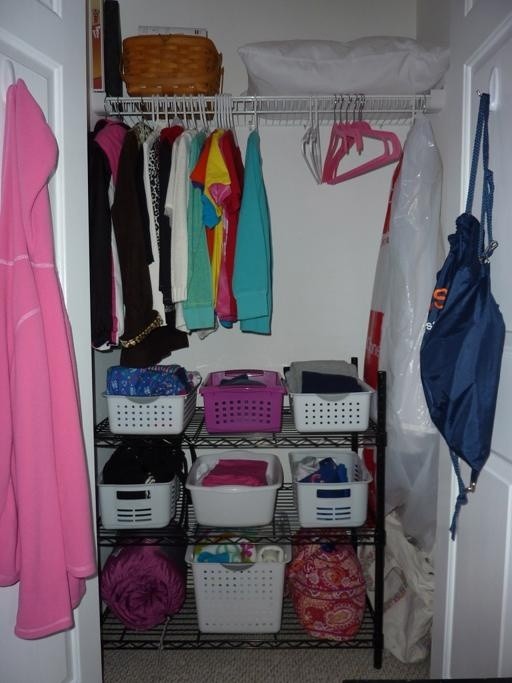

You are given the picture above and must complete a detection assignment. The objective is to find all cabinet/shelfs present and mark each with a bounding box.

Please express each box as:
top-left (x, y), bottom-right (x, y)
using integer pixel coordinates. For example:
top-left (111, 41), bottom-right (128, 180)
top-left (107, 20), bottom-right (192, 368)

top-left (94, 357), bottom-right (386, 669)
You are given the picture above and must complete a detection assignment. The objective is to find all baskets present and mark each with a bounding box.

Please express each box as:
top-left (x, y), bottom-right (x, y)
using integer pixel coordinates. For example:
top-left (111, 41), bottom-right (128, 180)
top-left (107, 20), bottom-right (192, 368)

top-left (101, 371), bottom-right (201, 436)
top-left (97, 476), bottom-right (181, 529)
top-left (199, 371), bottom-right (287, 434)
top-left (184, 538), bottom-right (293, 635)
top-left (118, 35), bottom-right (223, 121)
top-left (288, 450), bottom-right (372, 530)
top-left (282, 376), bottom-right (375, 434)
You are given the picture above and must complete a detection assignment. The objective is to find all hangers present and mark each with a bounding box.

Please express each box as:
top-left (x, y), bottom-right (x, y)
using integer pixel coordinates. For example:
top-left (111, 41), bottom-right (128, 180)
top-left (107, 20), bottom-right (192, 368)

top-left (103, 93), bottom-right (430, 185)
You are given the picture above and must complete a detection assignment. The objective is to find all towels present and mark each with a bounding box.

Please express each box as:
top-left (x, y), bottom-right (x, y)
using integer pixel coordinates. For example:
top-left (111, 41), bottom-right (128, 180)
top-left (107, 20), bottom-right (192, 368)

top-left (2, 80), bottom-right (93, 640)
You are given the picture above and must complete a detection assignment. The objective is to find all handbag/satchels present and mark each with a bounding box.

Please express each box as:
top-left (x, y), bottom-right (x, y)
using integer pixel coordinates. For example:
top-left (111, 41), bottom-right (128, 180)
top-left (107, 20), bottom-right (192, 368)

top-left (361, 510), bottom-right (436, 665)
top-left (288, 526), bottom-right (367, 641)
top-left (417, 213), bottom-right (505, 472)
top-left (106, 433), bottom-right (197, 527)
top-left (105, 365), bottom-right (190, 397)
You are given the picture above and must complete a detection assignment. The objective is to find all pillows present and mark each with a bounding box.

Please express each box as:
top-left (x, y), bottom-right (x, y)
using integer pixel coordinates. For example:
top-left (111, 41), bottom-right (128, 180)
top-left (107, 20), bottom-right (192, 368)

top-left (237, 35), bottom-right (453, 120)
